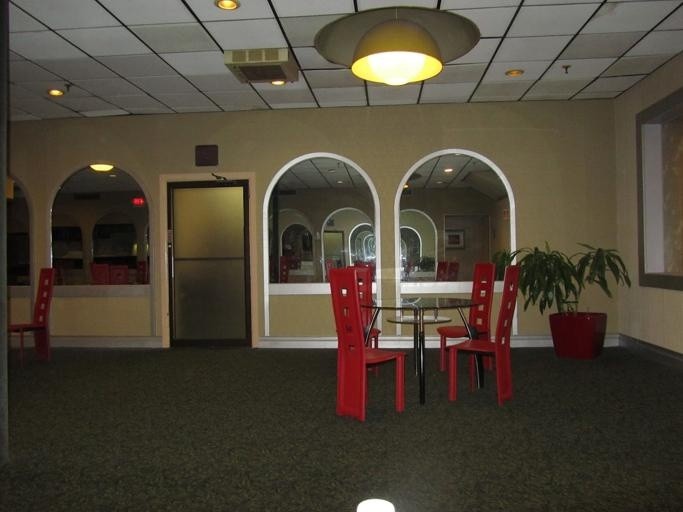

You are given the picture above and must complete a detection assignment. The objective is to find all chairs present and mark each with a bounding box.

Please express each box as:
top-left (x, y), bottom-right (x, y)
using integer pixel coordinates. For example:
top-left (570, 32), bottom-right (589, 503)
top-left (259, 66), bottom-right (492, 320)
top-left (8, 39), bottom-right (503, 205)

top-left (327, 262), bottom-right (520, 423)
top-left (7, 266), bottom-right (55, 366)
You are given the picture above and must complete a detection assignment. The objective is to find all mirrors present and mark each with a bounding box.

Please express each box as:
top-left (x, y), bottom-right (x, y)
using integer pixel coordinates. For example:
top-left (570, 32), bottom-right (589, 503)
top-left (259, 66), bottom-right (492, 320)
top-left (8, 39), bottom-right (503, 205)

top-left (635, 88), bottom-right (682, 290)
top-left (4, 162), bottom-right (151, 298)
top-left (260, 149), bottom-right (516, 295)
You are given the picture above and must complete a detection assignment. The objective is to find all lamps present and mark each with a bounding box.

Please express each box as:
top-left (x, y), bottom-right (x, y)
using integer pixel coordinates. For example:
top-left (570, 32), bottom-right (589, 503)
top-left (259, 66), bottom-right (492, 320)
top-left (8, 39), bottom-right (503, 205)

top-left (350, 18), bottom-right (446, 87)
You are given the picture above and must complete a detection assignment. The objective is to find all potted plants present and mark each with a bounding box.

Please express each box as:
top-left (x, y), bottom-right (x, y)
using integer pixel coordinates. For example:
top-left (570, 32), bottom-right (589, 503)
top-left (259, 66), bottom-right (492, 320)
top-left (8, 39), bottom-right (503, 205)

top-left (503, 236), bottom-right (632, 360)
